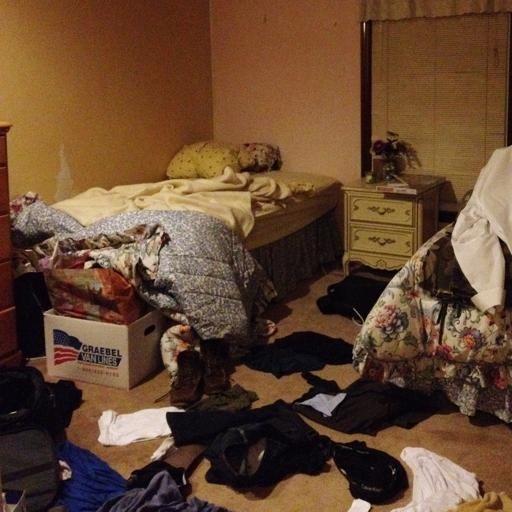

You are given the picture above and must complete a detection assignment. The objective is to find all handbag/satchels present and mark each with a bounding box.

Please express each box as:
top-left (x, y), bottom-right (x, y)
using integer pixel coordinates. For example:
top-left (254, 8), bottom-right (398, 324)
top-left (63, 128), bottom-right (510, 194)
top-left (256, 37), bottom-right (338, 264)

top-left (166, 410), bottom-right (237, 445)
top-left (292, 379), bottom-right (382, 434)
top-left (39, 256), bottom-right (145, 324)
top-left (318, 274), bottom-right (387, 321)
top-left (1, 426), bottom-right (60, 512)
top-left (334, 440), bottom-right (410, 505)
top-left (16, 271), bottom-right (50, 358)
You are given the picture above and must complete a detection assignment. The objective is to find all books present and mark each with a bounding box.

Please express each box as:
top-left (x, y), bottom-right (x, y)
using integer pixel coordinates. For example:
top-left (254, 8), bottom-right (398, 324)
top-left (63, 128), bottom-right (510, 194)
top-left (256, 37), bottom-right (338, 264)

top-left (375, 173), bottom-right (446, 195)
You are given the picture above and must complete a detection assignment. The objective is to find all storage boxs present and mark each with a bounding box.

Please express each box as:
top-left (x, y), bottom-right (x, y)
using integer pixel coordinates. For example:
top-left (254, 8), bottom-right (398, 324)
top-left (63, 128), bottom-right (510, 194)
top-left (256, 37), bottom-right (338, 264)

top-left (42, 307), bottom-right (163, 391)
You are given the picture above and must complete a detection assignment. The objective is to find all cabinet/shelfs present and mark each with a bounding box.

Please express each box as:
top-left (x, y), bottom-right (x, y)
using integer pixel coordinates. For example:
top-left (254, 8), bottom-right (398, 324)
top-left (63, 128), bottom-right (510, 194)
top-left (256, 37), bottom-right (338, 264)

top-left (0, 121), bottom-right (23, 373)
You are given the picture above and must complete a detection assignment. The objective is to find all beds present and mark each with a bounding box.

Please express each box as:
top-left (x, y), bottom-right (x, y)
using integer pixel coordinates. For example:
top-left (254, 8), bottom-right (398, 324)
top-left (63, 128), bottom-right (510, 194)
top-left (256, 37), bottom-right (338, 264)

top-left (11, 169), bottom-right (343, 321)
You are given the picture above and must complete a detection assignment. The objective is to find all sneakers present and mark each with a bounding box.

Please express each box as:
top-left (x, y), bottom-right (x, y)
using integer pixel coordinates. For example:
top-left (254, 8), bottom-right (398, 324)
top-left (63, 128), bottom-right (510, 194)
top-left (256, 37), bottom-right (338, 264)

top-left (199, 338), bottom-right (230, 393)
top-left (170, 349), bottom-right (203, 409)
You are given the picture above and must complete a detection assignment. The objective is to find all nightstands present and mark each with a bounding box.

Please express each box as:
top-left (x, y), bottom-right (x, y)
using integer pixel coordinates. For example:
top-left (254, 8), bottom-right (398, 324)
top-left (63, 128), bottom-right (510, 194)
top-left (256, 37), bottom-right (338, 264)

top-left (342, 175), bottom-right (446, 279)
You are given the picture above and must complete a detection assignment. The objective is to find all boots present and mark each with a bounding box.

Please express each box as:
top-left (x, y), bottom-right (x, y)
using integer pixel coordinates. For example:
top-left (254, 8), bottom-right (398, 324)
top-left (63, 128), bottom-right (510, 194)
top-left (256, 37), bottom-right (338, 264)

top-left (128, 461), bottom-right (191, 497)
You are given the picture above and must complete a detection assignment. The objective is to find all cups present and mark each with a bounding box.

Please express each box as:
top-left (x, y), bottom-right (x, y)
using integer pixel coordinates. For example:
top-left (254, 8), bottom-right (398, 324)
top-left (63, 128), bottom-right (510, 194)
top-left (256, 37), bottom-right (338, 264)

top-left (364, 171), bottom-right (377, 184)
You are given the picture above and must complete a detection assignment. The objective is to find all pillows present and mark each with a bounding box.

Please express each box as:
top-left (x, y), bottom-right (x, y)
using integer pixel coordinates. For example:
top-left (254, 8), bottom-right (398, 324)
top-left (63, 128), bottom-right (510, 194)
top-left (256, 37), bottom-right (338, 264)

top-left (166, 140), bottom-right (241, 179)
top-left (233, 143), bottom-right (280, 172)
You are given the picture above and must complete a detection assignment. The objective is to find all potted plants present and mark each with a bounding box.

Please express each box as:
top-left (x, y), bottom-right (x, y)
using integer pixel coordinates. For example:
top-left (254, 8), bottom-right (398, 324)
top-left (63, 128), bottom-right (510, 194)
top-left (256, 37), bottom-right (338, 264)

top-left (372, 129), bottom-right (409, 180)
